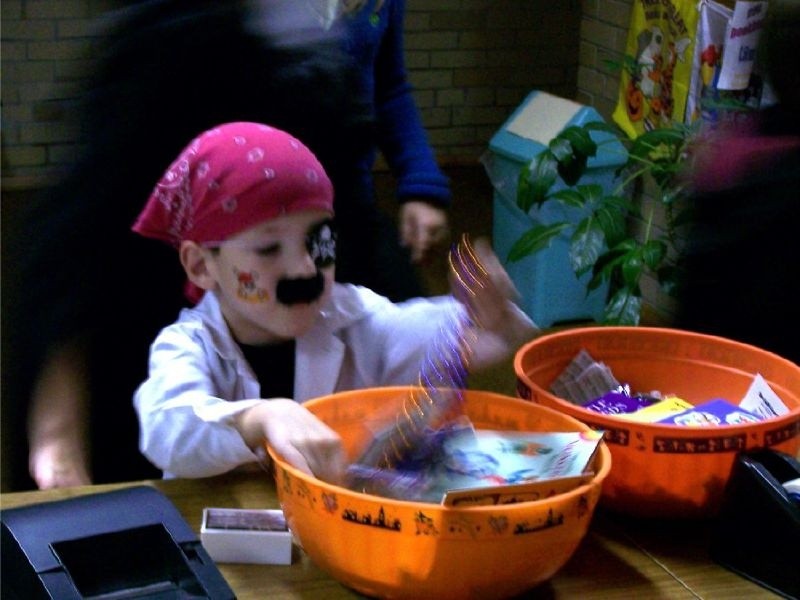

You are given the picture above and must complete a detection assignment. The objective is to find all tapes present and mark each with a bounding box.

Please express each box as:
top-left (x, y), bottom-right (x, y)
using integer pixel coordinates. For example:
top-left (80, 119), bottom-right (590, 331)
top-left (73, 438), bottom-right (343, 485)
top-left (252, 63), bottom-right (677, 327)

top-left (782, 478), bottom-right (800, 501)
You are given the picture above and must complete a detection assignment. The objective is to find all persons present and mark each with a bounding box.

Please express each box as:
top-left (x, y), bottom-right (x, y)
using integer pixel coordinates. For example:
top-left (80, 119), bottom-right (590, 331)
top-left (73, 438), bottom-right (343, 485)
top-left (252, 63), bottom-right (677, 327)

top-left (129, 122), bottom-right (544, 481)
top-left (14, 1), bottom-right (454, 493)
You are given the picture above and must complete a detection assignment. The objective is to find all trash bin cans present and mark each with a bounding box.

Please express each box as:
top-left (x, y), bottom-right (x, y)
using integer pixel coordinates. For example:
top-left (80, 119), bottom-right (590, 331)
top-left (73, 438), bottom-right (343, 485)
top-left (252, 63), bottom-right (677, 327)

top-left (489, 91), bottom-right (630, 328)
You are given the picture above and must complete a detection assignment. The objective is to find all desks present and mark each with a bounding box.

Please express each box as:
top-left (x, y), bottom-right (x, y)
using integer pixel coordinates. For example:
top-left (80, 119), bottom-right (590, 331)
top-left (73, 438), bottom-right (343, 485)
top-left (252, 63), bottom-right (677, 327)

top-left (0, 471), bottom-right (790, 600)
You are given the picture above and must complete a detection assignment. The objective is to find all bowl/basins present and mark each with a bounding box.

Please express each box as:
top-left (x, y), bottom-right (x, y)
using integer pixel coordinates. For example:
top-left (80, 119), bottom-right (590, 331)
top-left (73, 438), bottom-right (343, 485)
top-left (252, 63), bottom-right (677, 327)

top-left (263, 386), bottom-right (613, 600)
top-left (513, 327), bottom-right (800, 513)
